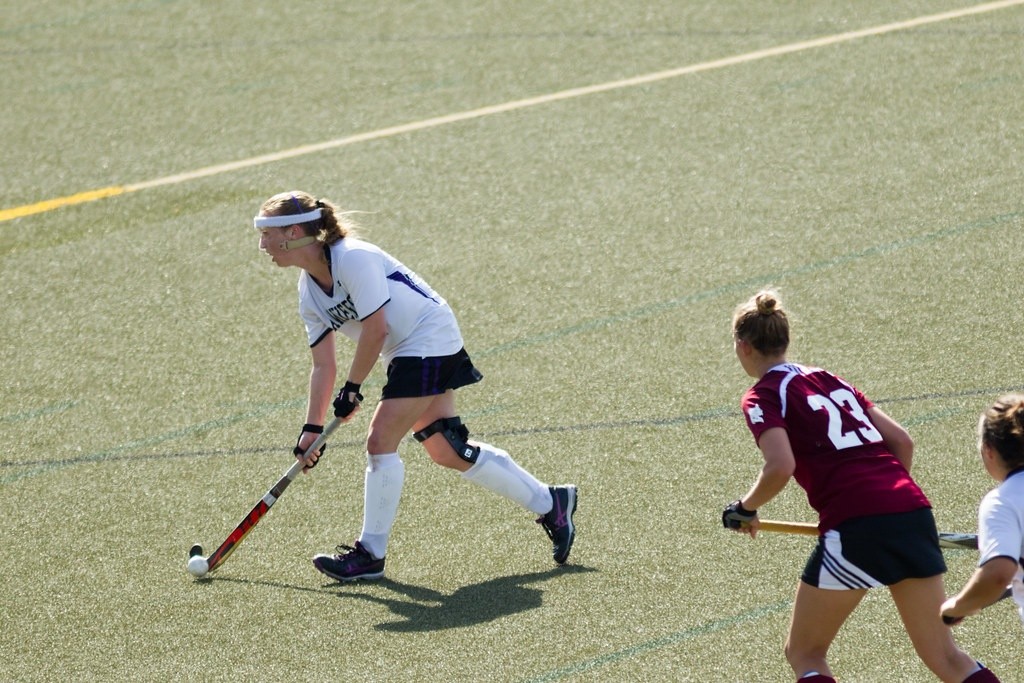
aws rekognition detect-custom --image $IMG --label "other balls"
[188,554,209,576]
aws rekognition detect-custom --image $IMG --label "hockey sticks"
[189,397,358,572]
[724,519,979,550]
[941,584,1013,624]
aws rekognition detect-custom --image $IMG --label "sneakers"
[535,484,578,564]
[313,539,385,583]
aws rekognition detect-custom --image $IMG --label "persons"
[254,190,578,582]
[940,391,1024,628]
[722,289,1000,683]
